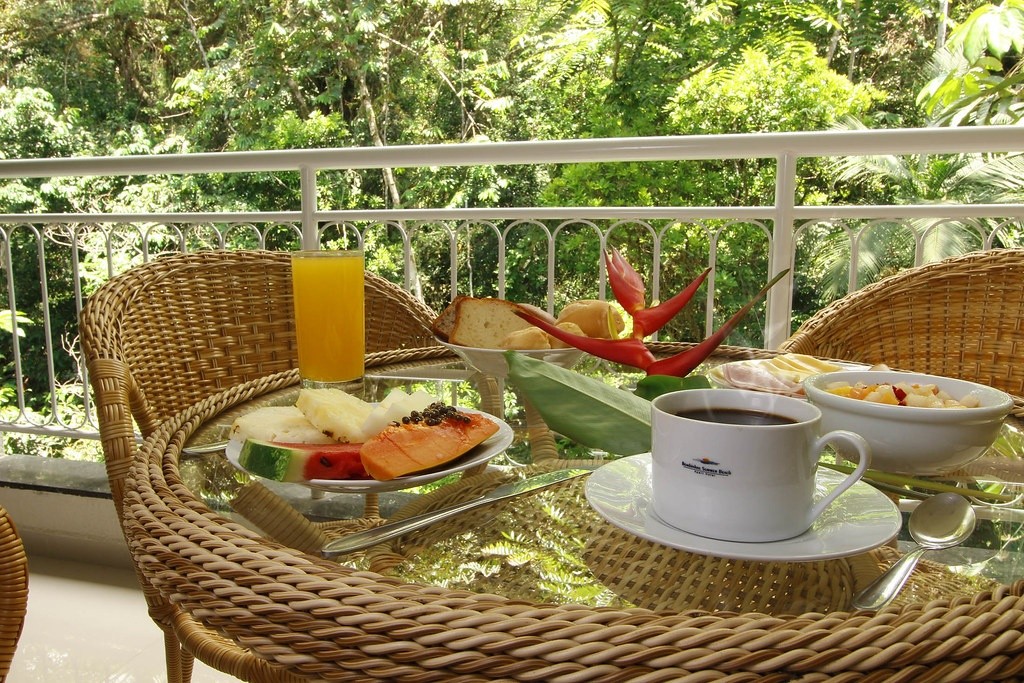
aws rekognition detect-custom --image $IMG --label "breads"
[451,294,537,351]
[554,300,624,338]
[432,293,467,340]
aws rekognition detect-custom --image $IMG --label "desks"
[130,338,1024,683]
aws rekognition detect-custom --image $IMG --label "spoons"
[852,492,976,612]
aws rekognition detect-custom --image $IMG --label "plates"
[583,452,902,562]
[709,359,872,395]
[226,402,514,493]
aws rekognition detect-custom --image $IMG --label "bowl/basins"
[432,337,584,375]
[802,371,1014,475]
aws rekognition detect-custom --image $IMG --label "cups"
[650,389,872,543]
[292,251,365,398]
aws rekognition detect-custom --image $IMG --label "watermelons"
[238,439,370,482]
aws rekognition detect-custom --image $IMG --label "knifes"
[322,468,594,553]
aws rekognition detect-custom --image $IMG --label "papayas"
[361,402,500,482]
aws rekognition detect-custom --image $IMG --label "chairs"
[79,248,507,683]
[777,248,1024,609]
